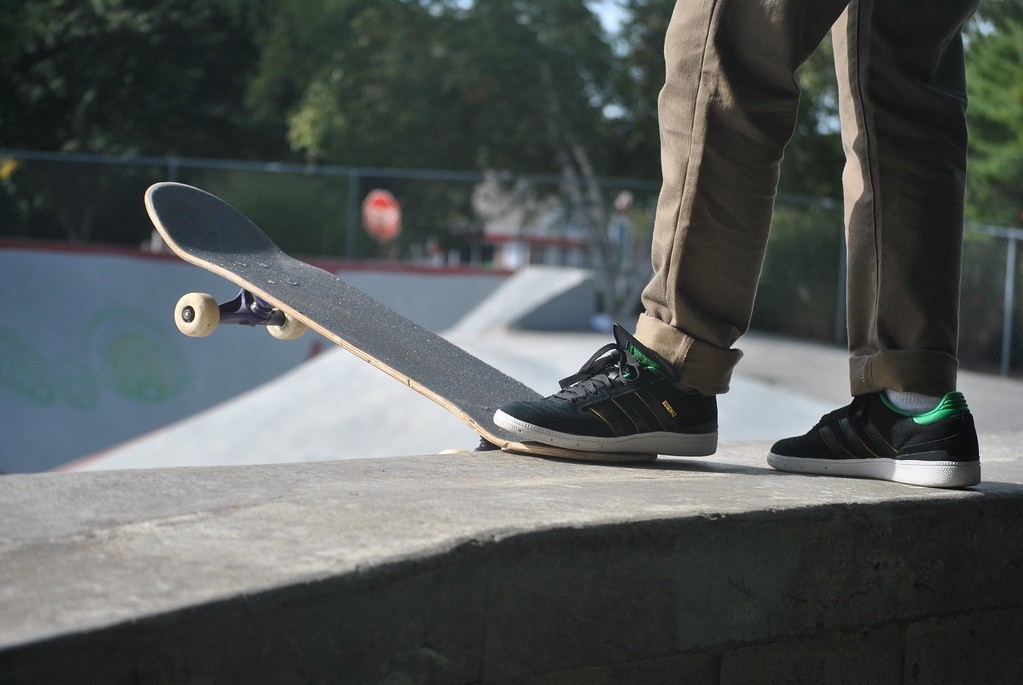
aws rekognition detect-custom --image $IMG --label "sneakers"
[768,390,981,487]
[492,322,718,457]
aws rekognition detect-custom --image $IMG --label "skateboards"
[144,180,658,466]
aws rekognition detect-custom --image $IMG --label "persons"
[493,0,989,489]
